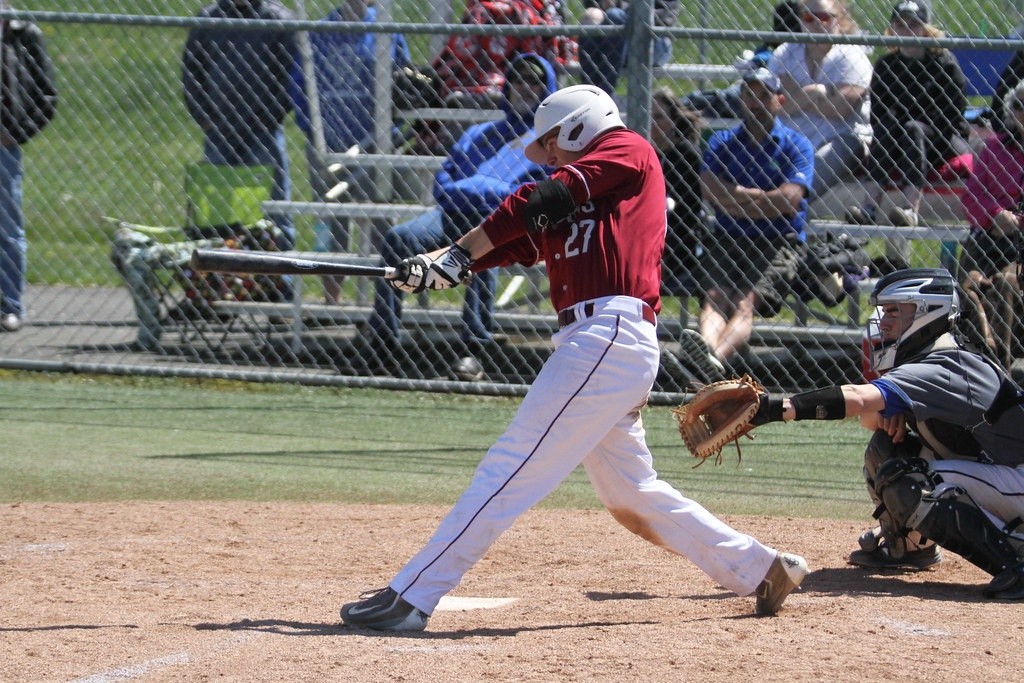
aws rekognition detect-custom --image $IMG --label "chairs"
[100,162,277,345]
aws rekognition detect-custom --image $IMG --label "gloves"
[390,254,433,295]
[425,244,475,290]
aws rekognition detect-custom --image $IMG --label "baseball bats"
[189,247,475,287]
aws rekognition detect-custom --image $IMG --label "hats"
[742,65,784,97]
[891,0,929,25]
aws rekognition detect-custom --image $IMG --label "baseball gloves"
[669,371,766,472]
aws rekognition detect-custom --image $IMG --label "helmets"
[868,266,961,374]
[522,83,629,163]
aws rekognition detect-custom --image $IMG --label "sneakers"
[341,587,427,633]
[756,552,811,617]
[848,530,941,572]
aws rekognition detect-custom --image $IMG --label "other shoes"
[680,328,726,381]
[0,313,22,331]
[844,205,874,224]
[658,349,704,394]
[336,351,402,376]
[889,205,918,227]
[450,351,485,383]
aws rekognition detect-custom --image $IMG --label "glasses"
[798,11,836,25]
[514,73,542,85]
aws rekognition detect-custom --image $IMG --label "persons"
[846,0,966,227]
[645,86,700,294]
[341,83,809,633]
[169,0,303,320]
[766,0,873,204]
[577,0,679,97]
[332,52,556,382]
[392,0,569,158]
[289,0,412,305]
[0,0,56,332]
[654,68,815,393]
[681,268,1024,598]
[956,80,1024,342]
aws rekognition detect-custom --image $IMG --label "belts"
[558,301,655,328]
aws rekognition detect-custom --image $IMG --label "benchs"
[209,61,972,346]
